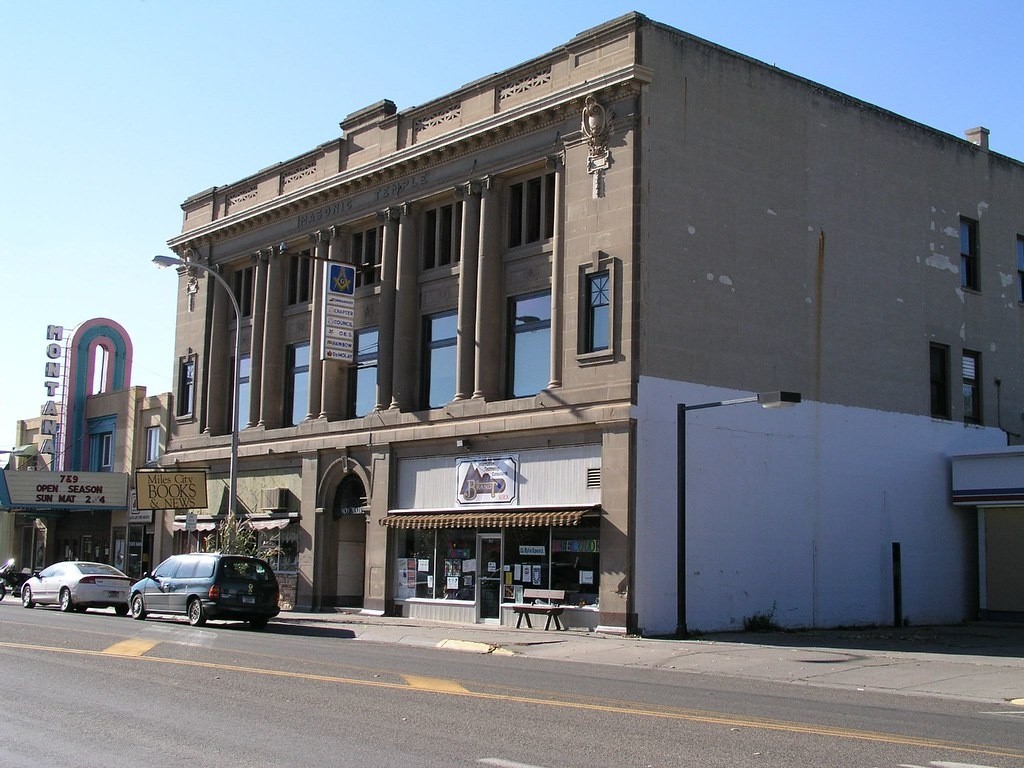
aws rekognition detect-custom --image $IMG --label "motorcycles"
[0,558,36,602]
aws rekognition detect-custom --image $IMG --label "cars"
[19,560,139,617]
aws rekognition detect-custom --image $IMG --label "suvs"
[127,550,282,632]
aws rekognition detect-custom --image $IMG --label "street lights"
[151,253,241,561]
[675,388,803,643]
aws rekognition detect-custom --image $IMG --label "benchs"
[513,607,564,631]
